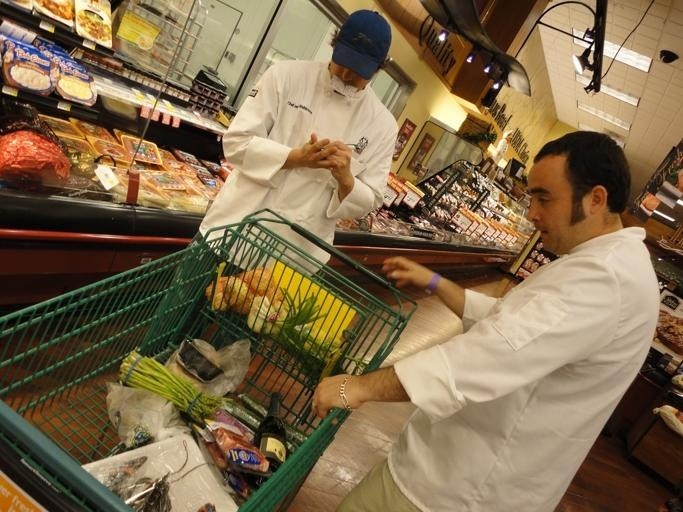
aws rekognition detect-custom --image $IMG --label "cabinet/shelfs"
[2,0,535,309]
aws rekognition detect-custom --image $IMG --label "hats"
[330,9,392,80]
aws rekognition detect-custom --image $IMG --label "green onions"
[255,287,327,357]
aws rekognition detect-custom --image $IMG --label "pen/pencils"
[321,143,360,150]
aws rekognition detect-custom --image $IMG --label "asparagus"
[118,350,224,425]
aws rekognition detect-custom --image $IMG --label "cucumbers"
[225,393,309,454]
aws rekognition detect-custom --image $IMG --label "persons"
[311,132,659,512]
[143,9,400,362]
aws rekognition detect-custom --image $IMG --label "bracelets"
[341,373,353,412]
[424,272,442,297]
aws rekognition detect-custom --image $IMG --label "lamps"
[570,0,609,95]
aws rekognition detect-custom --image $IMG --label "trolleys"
[0,206,419,511]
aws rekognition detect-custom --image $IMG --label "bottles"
[252,390,288,488]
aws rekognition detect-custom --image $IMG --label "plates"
[9,63,53,92]
[57,79,95,102]
